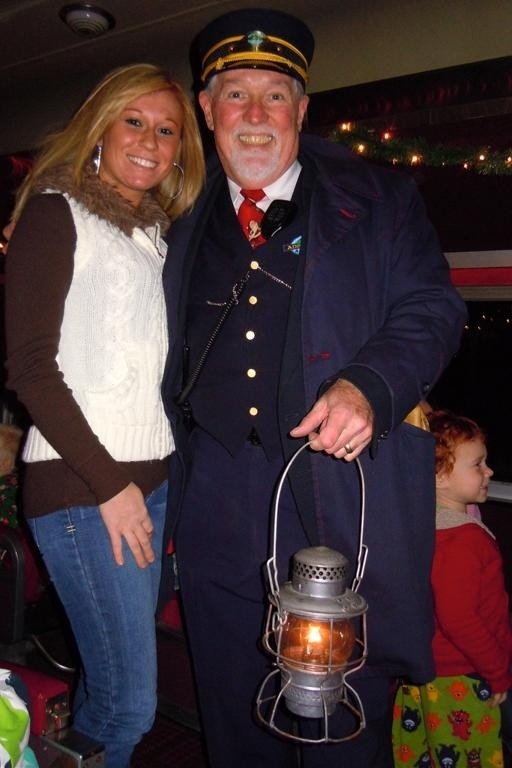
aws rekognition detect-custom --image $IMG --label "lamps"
[252,440,375,744]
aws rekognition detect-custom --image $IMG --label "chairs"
[0,513,205,768]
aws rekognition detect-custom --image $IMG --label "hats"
[197,8,314,91]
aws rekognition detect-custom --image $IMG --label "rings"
[343,443,352,455]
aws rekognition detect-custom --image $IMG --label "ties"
[235,189,266,249]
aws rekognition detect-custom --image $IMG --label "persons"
[4,57,205,765]
[1,8,469,767]
[422,407,509,712]
